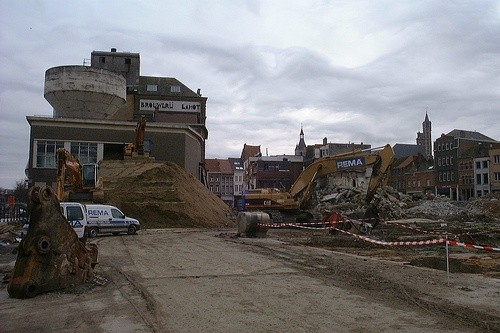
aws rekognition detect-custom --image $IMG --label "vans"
[83,204,140,237]
[21,202,88,246]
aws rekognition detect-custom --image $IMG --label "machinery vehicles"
[240,143,396,228]
[54,148,100,205]
[124,119,157,160]
